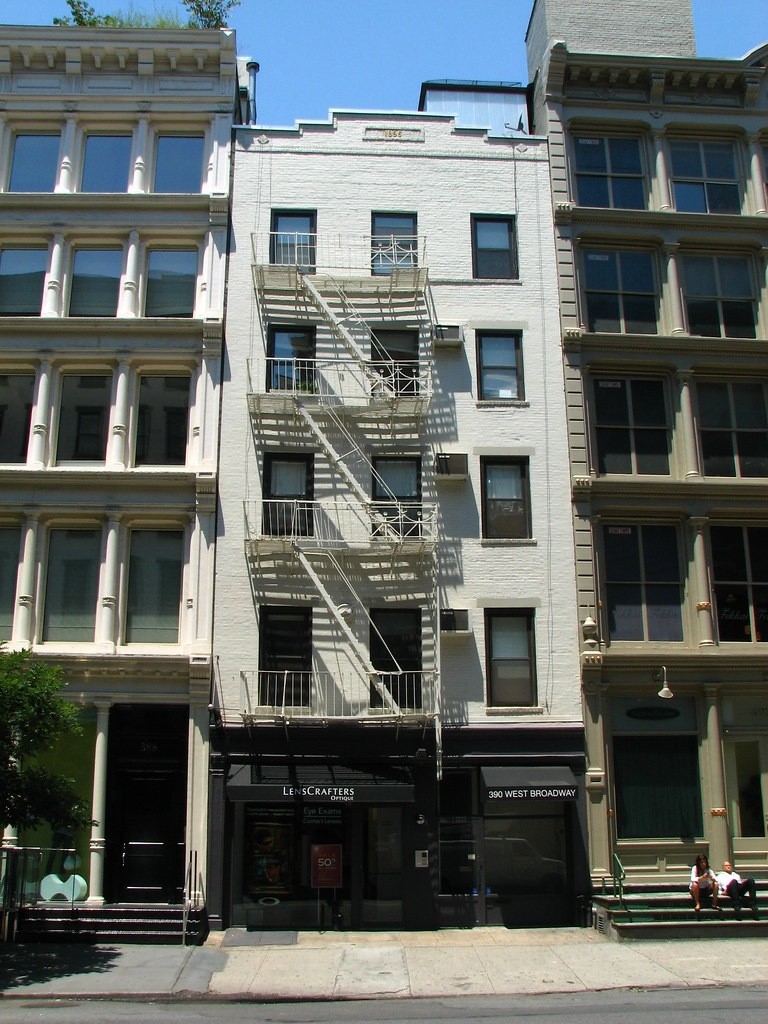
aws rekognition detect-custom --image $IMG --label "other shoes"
[750,903,759,911]
[712,905,720,909]
[734,904,742,912]
[695,905,700,911]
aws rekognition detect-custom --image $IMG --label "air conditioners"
[435,451,470,480]
[433,324,464,348]
[437,608,474,637]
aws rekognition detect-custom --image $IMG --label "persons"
[717,861,760,912]
[689,853,720,912]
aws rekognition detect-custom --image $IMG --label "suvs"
[438,837,568,897]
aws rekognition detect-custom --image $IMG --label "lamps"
[656,665,674,699]
